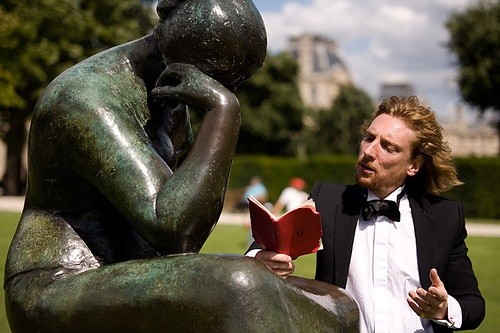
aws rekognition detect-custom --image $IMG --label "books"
[245,194,325,263]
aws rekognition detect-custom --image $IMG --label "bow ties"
[362,190,406,223]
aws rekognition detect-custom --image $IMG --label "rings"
[418,311,426,319]
[425,305,431,313]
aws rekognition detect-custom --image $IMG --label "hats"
[290,176,306,190]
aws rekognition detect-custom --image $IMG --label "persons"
[233,173,272,216]
[241,93,487,333]
[2,0,361,333]
[267,176,312,221]
